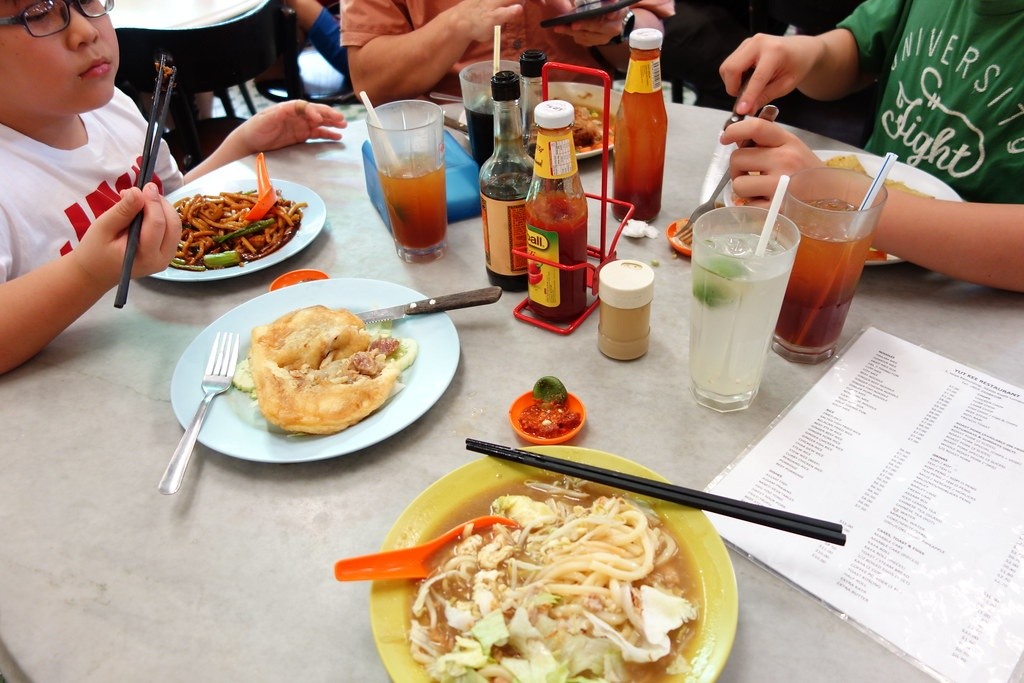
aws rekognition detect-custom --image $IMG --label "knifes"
[354,284,504,324]
[699,69,755,205]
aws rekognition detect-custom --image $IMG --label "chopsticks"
[113,54,178,308]
[465,437,847,545]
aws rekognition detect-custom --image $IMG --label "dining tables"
[0,102,1024,683]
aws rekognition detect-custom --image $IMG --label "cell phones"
[540,0,641,28]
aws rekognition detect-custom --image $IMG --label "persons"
[338,0,676,109]
[100,0,351,132]
[0,0,347,380]
[719,2,1023,295]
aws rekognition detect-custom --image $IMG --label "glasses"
[0,0,116,37]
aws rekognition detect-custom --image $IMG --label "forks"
[156,331,242,496]
[673,104,780,246]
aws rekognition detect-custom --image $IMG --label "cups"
[365,99,449,264]
[771,167,888,365]
[458,58,528,168]
[684,205,801,414]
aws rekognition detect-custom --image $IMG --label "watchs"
[613,10,635,44]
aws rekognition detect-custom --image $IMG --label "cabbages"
[425,585,698,683]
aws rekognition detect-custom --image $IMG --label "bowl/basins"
[369,444,738,683]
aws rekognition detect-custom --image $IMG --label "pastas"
[170,190,308,270]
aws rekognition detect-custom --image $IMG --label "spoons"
[335,515,521,584]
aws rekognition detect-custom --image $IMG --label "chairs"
[114,0,311,175]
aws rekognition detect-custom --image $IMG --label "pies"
[823,154,934,198]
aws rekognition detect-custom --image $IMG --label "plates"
[509,392,587,446]
[811,149,966,266]
[667,219,693,256]
[148,175,327,281]
[270,268,327,292]
[170,278,461,465]
[457,81,623,162]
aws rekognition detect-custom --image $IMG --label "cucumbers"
[363,319,419,374]
[232,359,256,392]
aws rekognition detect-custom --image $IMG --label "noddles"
[411,473,697,683]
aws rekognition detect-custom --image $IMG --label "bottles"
[475,27,667,364]
